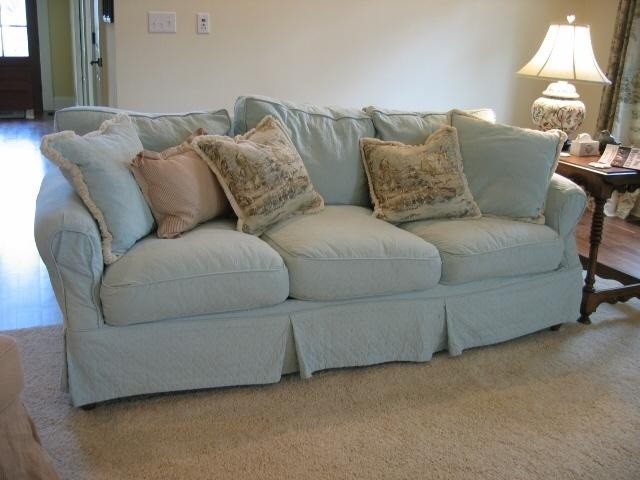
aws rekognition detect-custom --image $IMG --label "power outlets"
[197,12,209,35]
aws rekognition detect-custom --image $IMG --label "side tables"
[557,153,639,324]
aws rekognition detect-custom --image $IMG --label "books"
[598,144,640,170]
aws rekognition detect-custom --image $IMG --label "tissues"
[571,132,600,156]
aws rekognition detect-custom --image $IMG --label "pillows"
[359,124,481,226]
[192,114,324,238]
[130,128,231,239]
[41,113,156,266]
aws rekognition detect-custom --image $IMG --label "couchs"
[35,94,590,411]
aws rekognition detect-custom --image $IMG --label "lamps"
[516,13,612,144]
